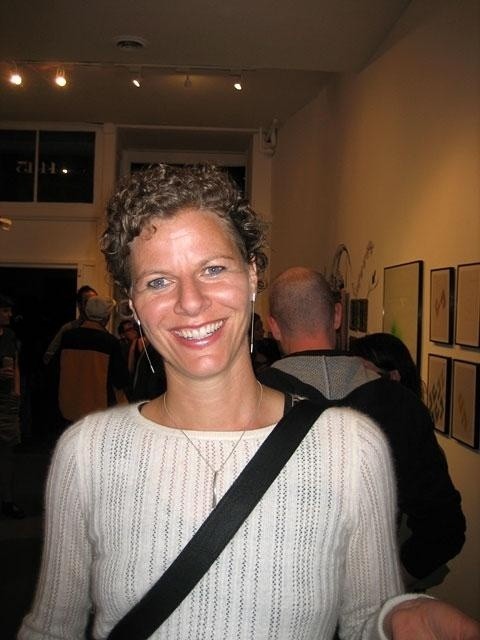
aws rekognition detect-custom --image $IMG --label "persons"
[0,296,26,520]
[15,160,480,639]
[348,332,449,594]
[249,313,280,374]
[253,266,466,593]
[40,284,168,433]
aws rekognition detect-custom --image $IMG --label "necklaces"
[163,377,264,509]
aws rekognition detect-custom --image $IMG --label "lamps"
[2,57,251,96]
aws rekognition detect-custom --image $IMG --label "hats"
[85,295,116,321]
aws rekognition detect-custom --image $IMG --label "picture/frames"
[380,259,423,380]
[450,358,479,453]
[427,351,451,434]
[454,263,480,353]
[428,265,455,348]
